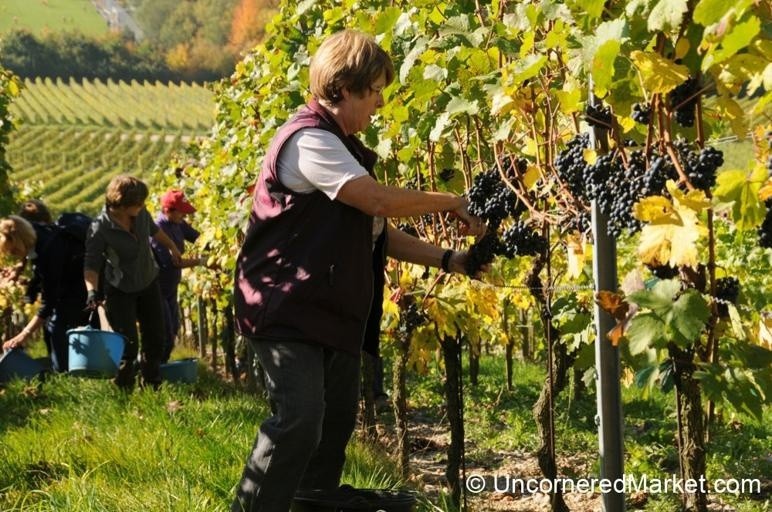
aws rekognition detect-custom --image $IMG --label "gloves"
[86,290,99,311]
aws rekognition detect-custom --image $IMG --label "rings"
[478,221,482,226]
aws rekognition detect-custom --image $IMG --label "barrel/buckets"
[0,347,49,385]
[160,358,201,386]
[64,310,128,379]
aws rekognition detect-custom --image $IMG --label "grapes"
[398,77,772,334]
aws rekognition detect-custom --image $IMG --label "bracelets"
[441,249,452,273]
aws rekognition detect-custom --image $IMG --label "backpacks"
[40,212,91,273]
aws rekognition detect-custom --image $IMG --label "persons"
[0,215,84,374]
[139,189,223,364]
[231,31,498,511]
[83,176,184,408]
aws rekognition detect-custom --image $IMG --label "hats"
[160,190,196,214]
